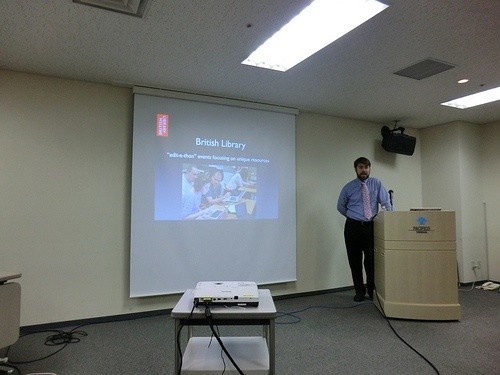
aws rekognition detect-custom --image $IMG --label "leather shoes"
[367,287,374,300]
[353,290,365,301]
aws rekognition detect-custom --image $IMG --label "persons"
[182,163,250,220]
[337,157,392,302]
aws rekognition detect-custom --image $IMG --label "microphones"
[388,189,393,206]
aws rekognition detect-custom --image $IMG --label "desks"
[0,270,21,350]
[237,172,256,199]
[170,287,278,375]
[192,198,256,218]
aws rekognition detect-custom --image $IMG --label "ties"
[360,182,372,220]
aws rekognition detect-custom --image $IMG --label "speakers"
[382,133,416,156]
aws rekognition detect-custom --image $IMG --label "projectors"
[193,281,259,308]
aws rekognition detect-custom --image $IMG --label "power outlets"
[473,264,483,273]
